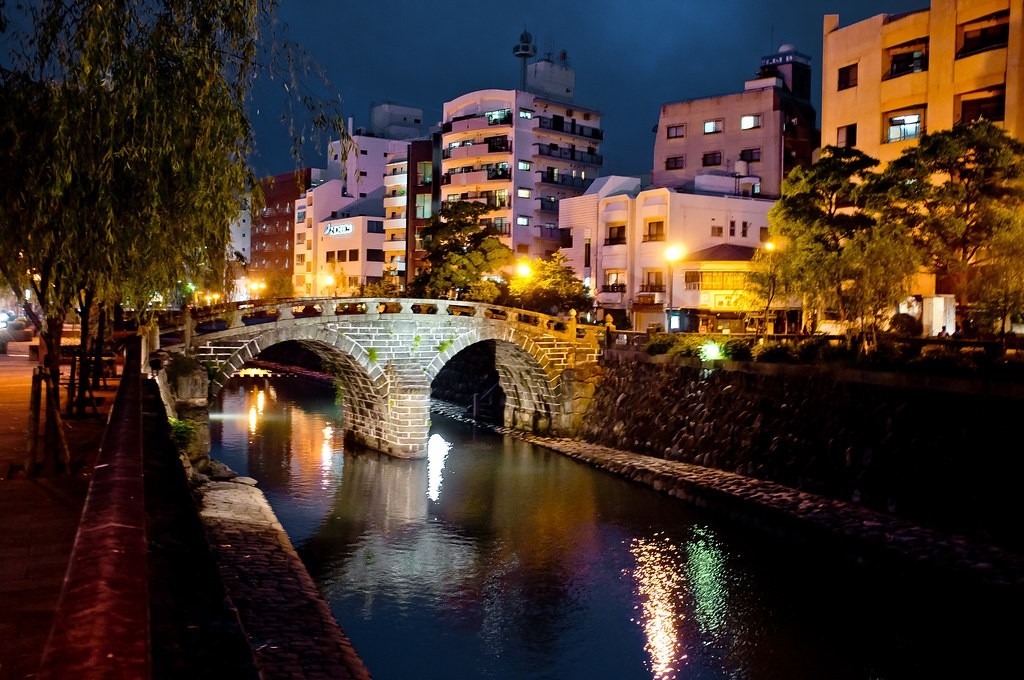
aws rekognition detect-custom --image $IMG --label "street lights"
[765,242,775,341]
[325,276,333,299]
[518,262,531,319]
[197,279,267,305]
[666,245,682,331]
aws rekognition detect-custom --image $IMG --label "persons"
[787,322,796,334]
[955,320,961,334]
[937,325,950,348]
